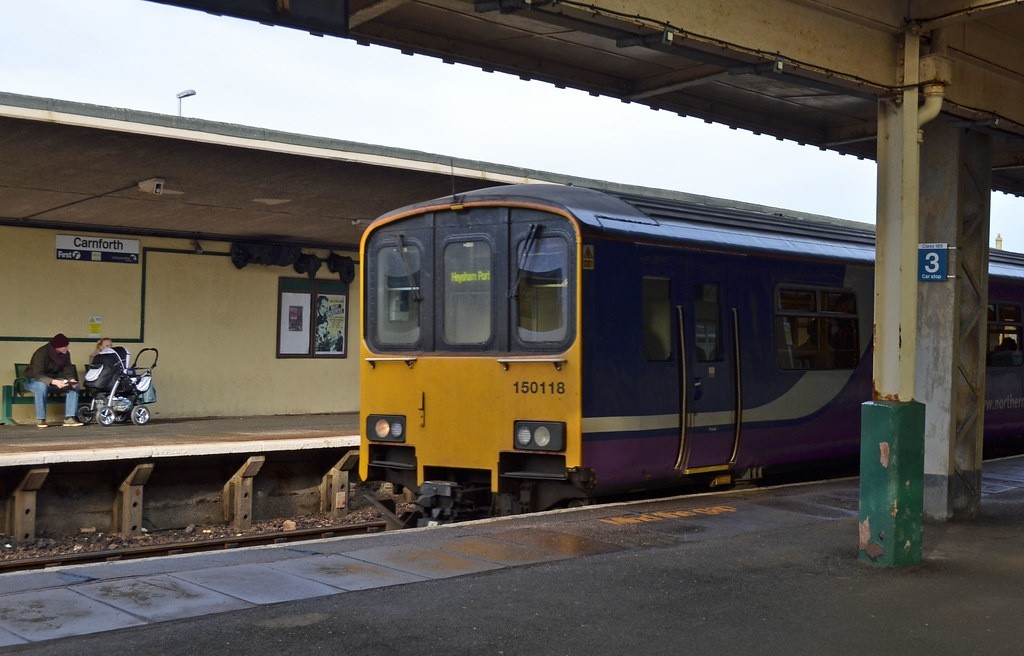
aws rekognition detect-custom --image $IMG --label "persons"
[23,333,84,428]
[88,338,113,364]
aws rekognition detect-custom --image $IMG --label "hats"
[51,333,68,347]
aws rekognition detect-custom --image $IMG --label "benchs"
[0,363,94,427]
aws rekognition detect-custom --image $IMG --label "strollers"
[74,344,159,427]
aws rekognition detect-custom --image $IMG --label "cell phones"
[65,382,77,385]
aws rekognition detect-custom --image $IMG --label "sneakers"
[62,418,83,427]
[37,420,49,428]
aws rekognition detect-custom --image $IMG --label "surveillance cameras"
[138,178,165,195]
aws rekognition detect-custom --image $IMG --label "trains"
[359,157,1024,513]
